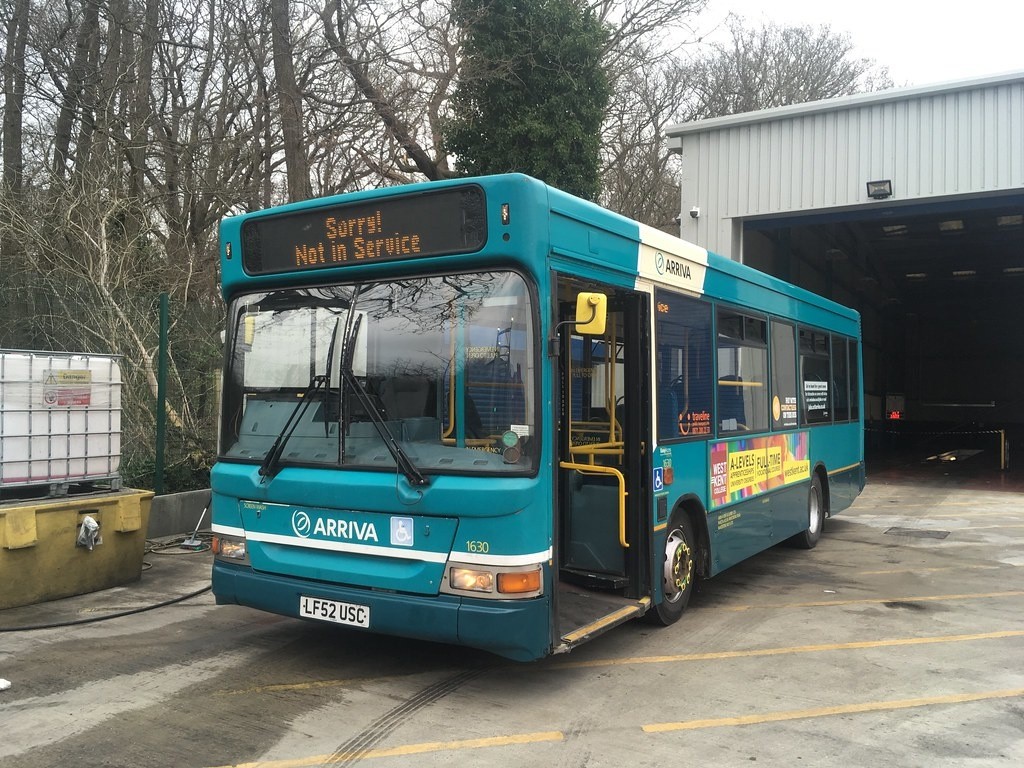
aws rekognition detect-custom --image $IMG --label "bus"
[213,173,864,663]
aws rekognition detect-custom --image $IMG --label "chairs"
[585,371,839,465]
[442,343,527,436]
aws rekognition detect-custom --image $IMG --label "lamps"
[866,179,893,200]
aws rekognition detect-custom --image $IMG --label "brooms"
[178,492,212,551]
